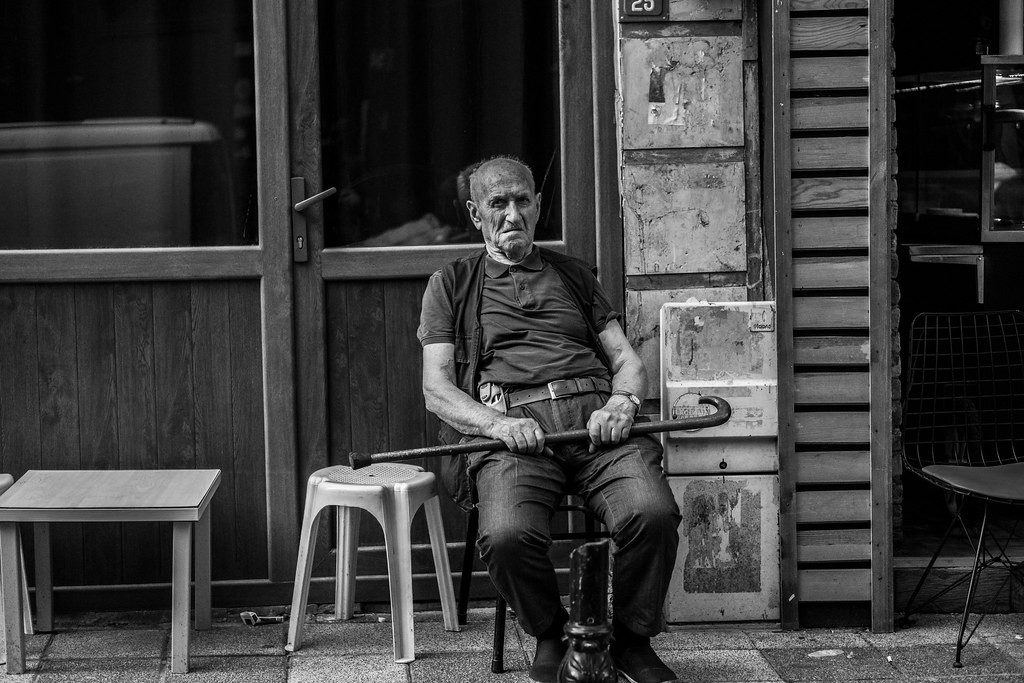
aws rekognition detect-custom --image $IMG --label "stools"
[284,463,460,663]
[460,484,610,673]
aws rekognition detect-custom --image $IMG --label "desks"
[0,469,225,675]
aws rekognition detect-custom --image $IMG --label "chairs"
[903,307,1024,670]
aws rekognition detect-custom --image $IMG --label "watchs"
[611,389,641,413]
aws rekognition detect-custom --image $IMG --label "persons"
[417,153,683,683]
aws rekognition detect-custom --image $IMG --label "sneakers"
[528,625,572,683]
[609,620,677,683]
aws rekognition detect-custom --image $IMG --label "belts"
[504,377,614,408]
